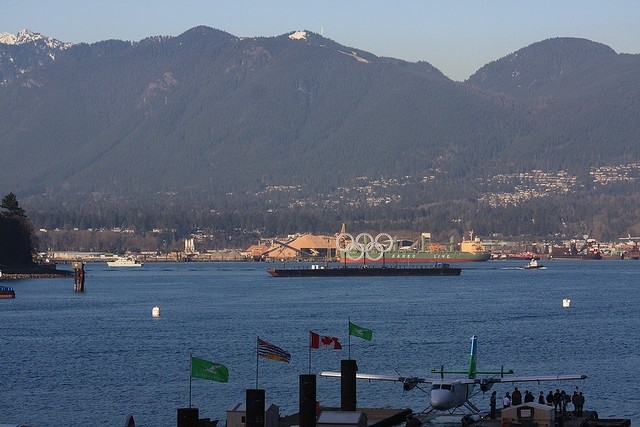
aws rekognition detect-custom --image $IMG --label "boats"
[1,286,16,299]
[107,254,144,267]
[524,259,543,269]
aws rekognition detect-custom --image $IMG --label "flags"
[258,338,291,363]
[311,332,342,350]
[191,357,229,382]
[349,321,372,341]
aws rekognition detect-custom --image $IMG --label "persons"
[489,391,497,419]
[571,391,579,412]
[512,387,522,405]
[553,389,562,413]
[539,391,546,405]
[561,389,567,413]
[524,390,535,403]
[503,395,511,409]
[546,391,554,407]
[578,392,585,415]
[504,392,511,401]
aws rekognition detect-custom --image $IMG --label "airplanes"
[320,334,587,427]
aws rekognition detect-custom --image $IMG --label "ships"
[266,267,461,277]
[338,235,492,263]
